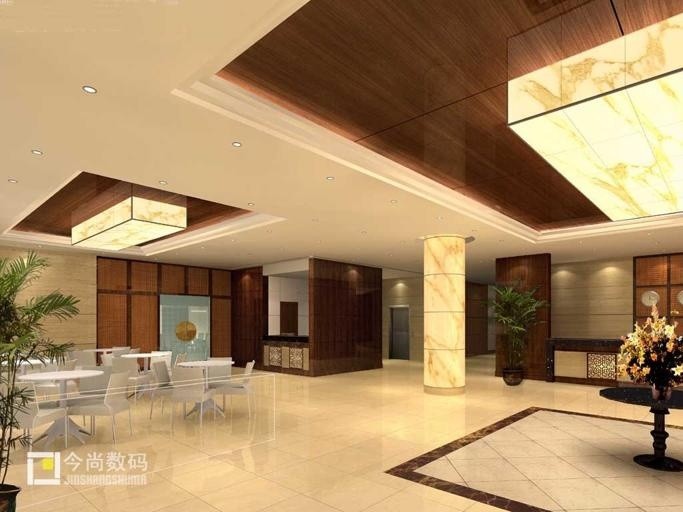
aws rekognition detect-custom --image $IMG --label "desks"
[600,387,683,471]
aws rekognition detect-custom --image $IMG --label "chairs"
[6,346,256,452]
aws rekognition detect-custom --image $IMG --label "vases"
[651,384,671,401]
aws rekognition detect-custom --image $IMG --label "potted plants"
[0,249,81,512]
[483,279,550,386]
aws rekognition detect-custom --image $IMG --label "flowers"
[615,303,683,389]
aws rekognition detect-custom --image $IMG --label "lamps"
[71,196,187,252]
[506,12,682,221]
